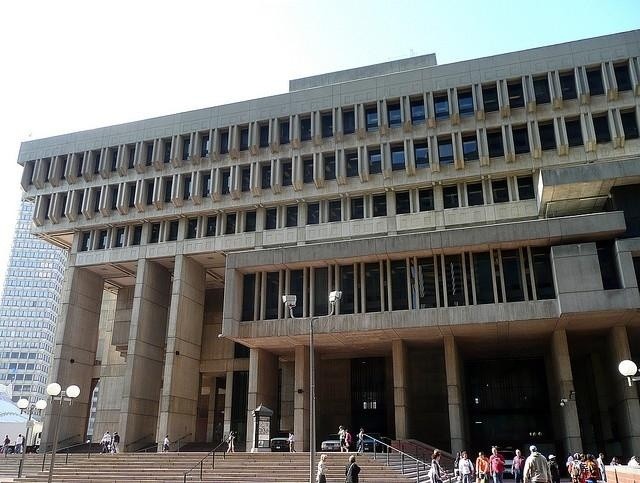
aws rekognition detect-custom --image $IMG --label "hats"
[548,454,557,460]
[529,445,537,453]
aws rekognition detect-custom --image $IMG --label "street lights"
[282,291,344,482]
[47,381,82,483]
[17,397,47,477]
[618,359,640,387]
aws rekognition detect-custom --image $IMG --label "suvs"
[497,450,518,477]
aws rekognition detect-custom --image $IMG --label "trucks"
[0,421,44,454]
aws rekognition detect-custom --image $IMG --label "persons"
[337,425,349,452]
[357,427,364,452]
[13,434,23,454]
[288,432,297,452]
[345,454,361,483]
[98,430,120,454]
[1,434,10,452]
[163,434,170,452]
[345,429,351,451]
[428,444,640,482]
[226,430,235,453]
[316,453,329,483]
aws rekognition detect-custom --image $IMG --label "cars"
[355,432,383,452]
[270,437,290,451]
[321,433,341,451]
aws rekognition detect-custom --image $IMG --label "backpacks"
[549,463,557,478]
[568,460,600,480]
